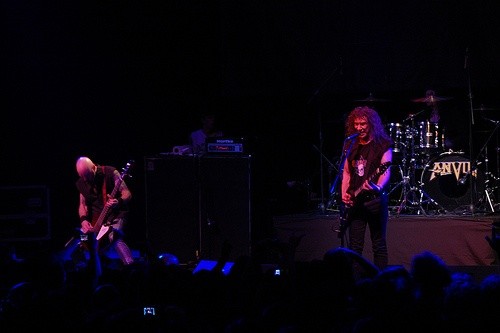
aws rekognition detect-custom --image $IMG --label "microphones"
[346,132,362,140]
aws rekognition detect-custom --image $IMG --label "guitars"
[91,162,133,240]
[338,160,393,237]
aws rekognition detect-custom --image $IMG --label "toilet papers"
[173,145,186,154]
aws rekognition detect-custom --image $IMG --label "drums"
[385,121,412,153]
[406,149,486,210]
[409,122,439,149]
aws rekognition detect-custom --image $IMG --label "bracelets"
[80,216,87,222]
[118,198,123,206]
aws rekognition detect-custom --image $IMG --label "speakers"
[139,154,251,264]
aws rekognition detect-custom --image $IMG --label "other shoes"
[96,283,105,298]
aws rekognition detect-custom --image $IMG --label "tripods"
[388,43,500,217]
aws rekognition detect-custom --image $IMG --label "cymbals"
[473,107,497,110]
[353,97,387,101]
[411,95,448,102]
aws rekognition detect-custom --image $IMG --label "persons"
[340,105,394,266]
[409,87,446,129]
[0,227,500,333]
[76,156,133,264]
